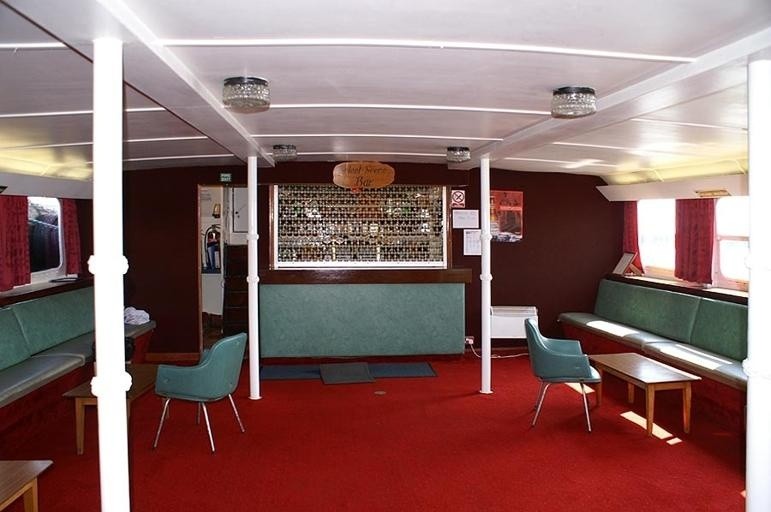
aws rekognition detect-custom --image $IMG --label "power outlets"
[465,335,475,345]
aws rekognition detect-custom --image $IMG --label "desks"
[582,349,704,440]
[0,458,54,511]
[58,360,170,455]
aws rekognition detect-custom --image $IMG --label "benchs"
[638,296,748,434]
[8,284,157,383]
[552,275,703,389]
[0,306,83,409]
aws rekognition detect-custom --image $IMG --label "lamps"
[221,74,272,115]
[272,144,298,163]
[445,144,472,165]
[549,85,599,121]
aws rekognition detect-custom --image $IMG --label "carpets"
[318,361,377,387]
[259,359,439,382]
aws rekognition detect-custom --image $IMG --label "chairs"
[522,316,604,434]
[149,331,249,455]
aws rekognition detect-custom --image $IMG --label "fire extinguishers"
[204,224,221,274]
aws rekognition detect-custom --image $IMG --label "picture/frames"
[611,251,639,276]
